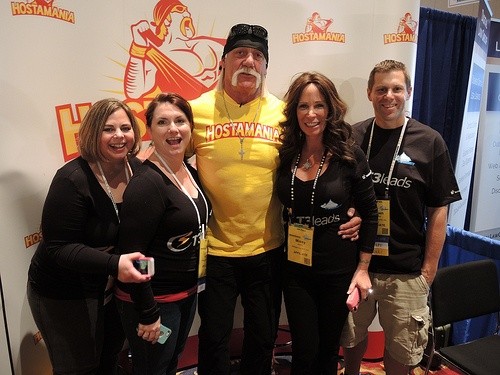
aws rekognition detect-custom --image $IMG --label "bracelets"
[359,259,370,265]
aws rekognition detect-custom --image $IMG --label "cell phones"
[346,286,361,312]
[132,256,155,278]
[148,324,172,345]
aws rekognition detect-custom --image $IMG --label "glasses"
[230,23,268,39]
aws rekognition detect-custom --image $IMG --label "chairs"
[425,259,500,375]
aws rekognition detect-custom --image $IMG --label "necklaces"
[222,88,264,159]
[96,158,133,224]
[302,155,311,171]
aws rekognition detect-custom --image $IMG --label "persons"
[27,99,151,375]
[351,60,462,375]
[143,23,361,375]
[274,70,378,375]
[112,93,212,375]
